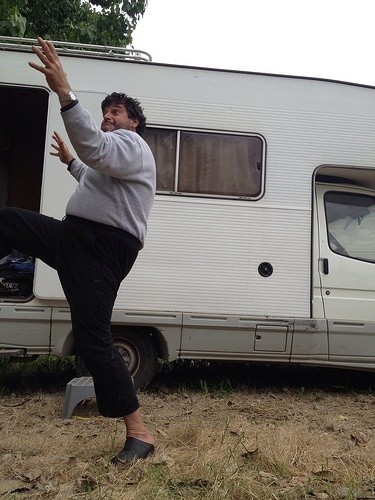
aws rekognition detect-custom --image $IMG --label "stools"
[61,376,96,419]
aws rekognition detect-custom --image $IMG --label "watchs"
[59,90,76,101]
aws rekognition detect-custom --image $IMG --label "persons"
[0,37,156,464]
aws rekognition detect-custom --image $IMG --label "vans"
[0,35,375,391]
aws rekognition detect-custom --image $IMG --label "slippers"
[111,436,155,466]
